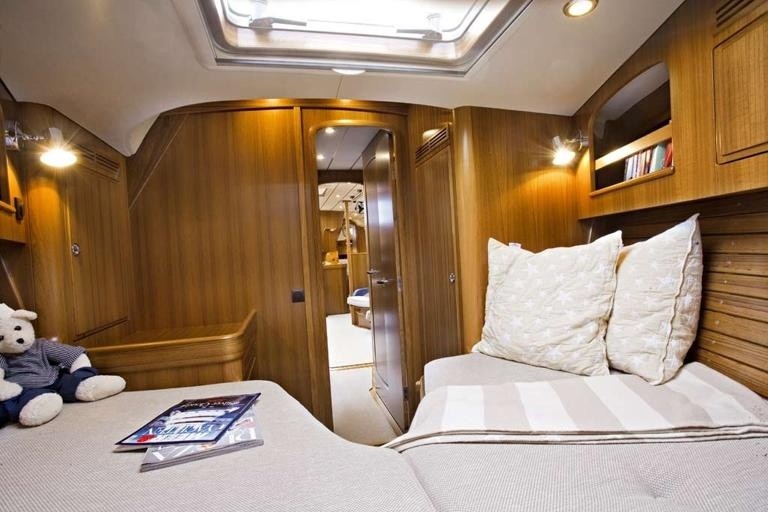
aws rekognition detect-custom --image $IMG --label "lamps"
[6,118,78,168]
[551,129,589,166]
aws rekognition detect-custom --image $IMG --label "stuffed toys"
[0,303,125,427]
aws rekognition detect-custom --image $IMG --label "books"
[115,393,260,452]
[623,139,672,182]
[140,403,264,472]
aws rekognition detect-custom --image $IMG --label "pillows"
[603,212,704,386]
[471,229,623,376]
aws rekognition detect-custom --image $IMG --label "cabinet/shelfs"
[572,0,768,221]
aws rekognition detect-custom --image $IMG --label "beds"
[0,378,435,512]
[401,352,768,512]
